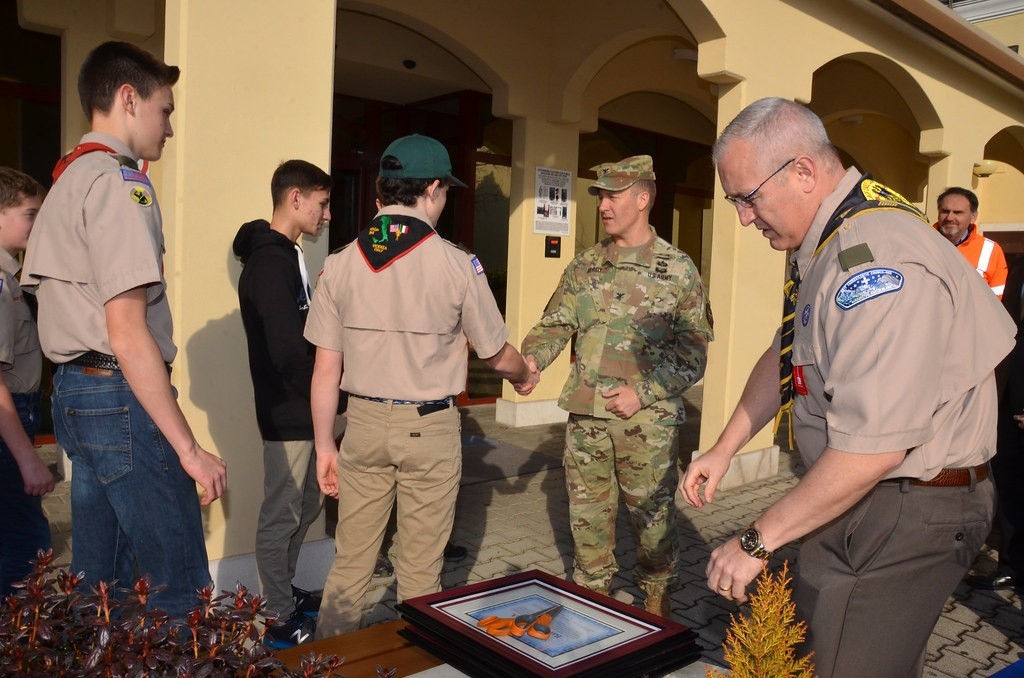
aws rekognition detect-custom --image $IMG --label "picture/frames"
[394,569,705,678]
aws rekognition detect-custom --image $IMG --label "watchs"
[740,521,773,561]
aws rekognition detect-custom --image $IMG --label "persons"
[232,160,338,650]
[679,96,1018,678]
[519,156,715,620]
[963,253,1024,600]
[933,186,1006,305]
[21,41,228,632]
[303,134,541,638]
[0,167,56,602]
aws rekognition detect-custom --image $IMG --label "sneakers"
[263,607,317,650]
[291,583,322,617]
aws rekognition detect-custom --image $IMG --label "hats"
[379,133,470,188]
[586,155,656,197]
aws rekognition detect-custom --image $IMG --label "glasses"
[724,159,795,208]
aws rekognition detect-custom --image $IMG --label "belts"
[347,390,457,417]
[66,351,173,375]
[876,464,992,487]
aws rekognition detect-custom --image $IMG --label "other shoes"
[966,545,1000,590]
[442,542,468,561]
[642,593,674,619]
[371,551,394,577]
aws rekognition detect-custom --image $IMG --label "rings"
[718,585,731,591]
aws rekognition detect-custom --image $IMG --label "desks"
[241,618,731,678]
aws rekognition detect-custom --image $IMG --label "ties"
[772,173,931,453]
[52,143,118,184]
[357,215,435,272]
